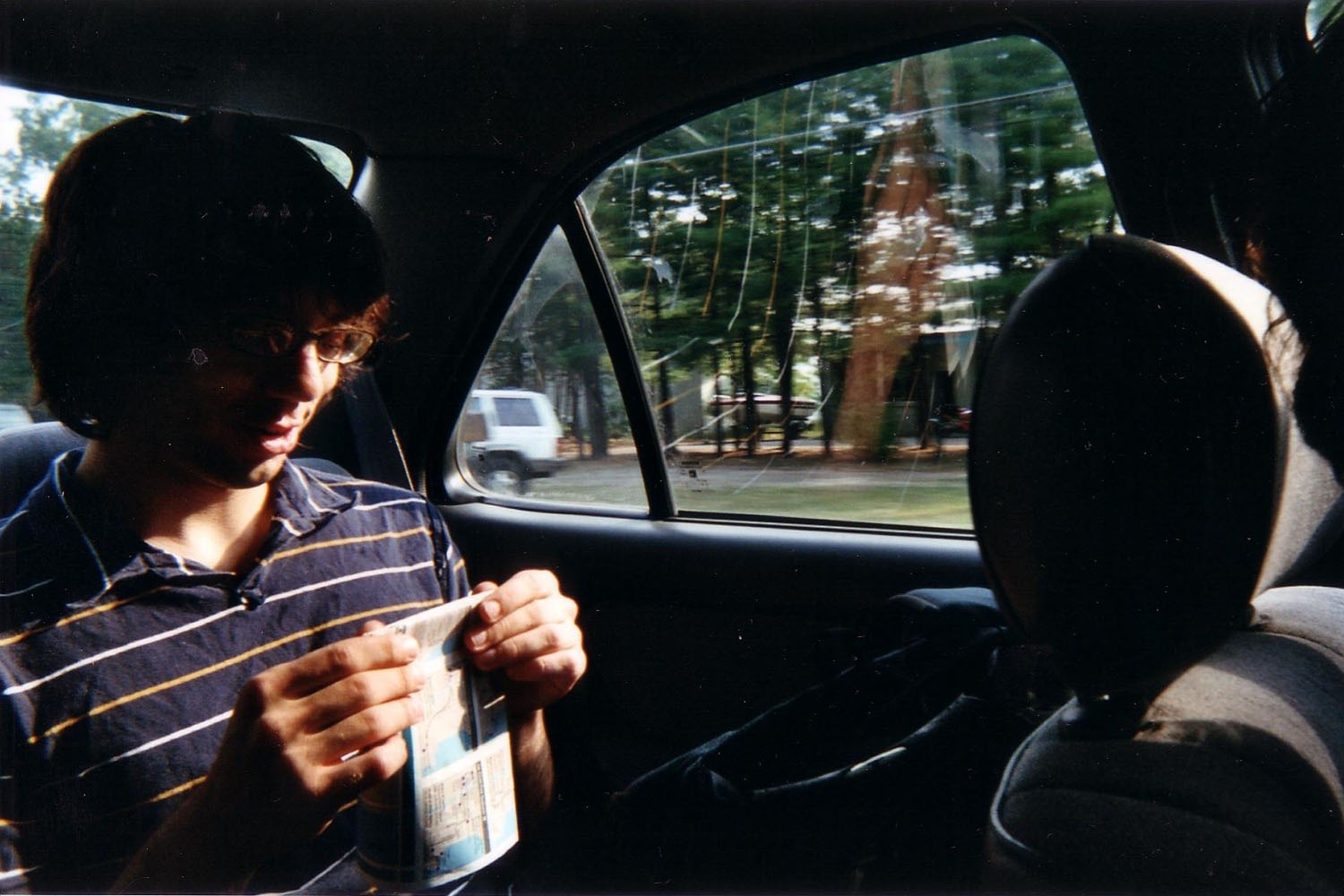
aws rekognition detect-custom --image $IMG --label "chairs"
[966,236,1344,896]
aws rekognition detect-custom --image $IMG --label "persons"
[1229,1,1344,487]
[0,112,587,895]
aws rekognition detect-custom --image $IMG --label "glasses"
[235,320,377,366]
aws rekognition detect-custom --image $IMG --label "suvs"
[460,389,568,497]
